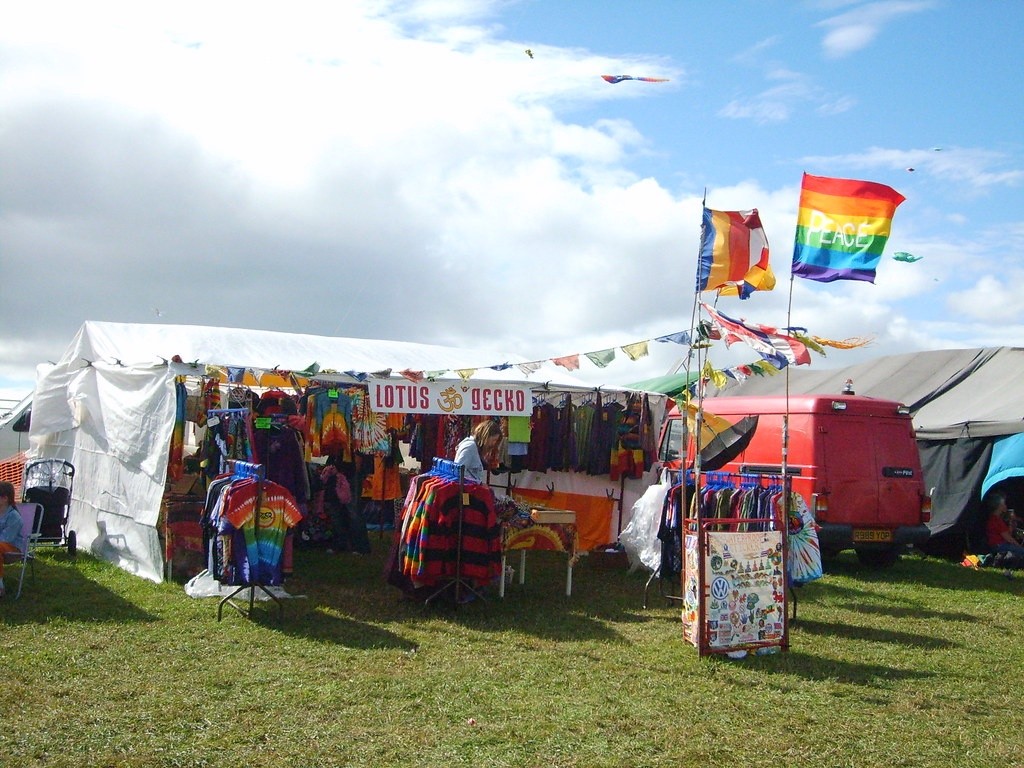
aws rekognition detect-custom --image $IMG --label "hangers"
[426,456,477,486]
[228,458,269,484]
[673,468,793,494]
[532,388,650,411]
[176,375,368,431]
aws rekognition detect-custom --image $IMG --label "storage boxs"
[528,505,577,523]
[505,568,514,584]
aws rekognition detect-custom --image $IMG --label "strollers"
[18,459,79,555]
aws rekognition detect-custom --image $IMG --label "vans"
[656,395,934,568]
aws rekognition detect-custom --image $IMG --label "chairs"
[3,502,45,600]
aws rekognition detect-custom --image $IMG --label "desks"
[498,519,577,598]
[154,499,207,584]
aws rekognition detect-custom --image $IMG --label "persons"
[984,490,1020,549]
[0,481,23,597]
[454,420,503,484]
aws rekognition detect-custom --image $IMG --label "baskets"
[505,565,515,584]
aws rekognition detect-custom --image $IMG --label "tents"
[621,346,1024,563]
[0,392,33,462]
[25,321,668,583]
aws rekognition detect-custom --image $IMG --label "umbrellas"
[687,414,758,471]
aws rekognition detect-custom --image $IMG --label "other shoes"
[0,577,4,589]
[1004,551,1013,560]
[994,552,1004,560]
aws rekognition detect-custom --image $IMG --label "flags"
[696,208,776,300]
[792,173,906,284]
